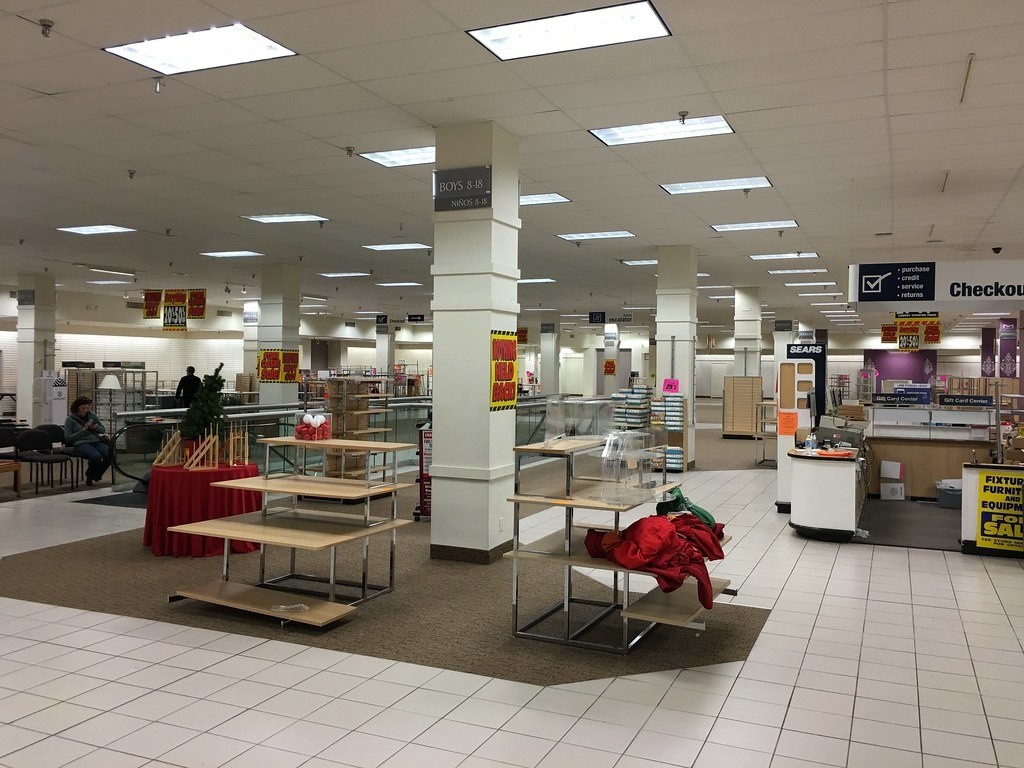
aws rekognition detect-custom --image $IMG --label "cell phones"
[92,422,99,425]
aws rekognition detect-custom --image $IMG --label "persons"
[176,366,203,408]
[299,370,303,399]
[306,374,314,401]
[65,396,117,486]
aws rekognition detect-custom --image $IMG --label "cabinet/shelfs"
[864,406,997,442]
[1001,395,1024,421]
[753,401,777,470]
[299,360,432,481]
[995,382,1024,465]
[947,377,1005,397]
[651,399,688,471]
[166,433,415,631]
[65,370,158,443]
[830,368,877,403]
[502,435,737,656]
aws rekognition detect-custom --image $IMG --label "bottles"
[811,433,816,449]
[805,435,812,456]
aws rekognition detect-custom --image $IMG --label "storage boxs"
[236,372,259,403]
[295,413,333,441]
[936,485,962,509]
[952,380,1024,465]
[611,385,684,471]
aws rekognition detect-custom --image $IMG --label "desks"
[218,391,258,398]
[143,461,263,558]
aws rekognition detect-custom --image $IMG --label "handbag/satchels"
[100,435,111,446]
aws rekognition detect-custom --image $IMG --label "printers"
[816,416,865,448]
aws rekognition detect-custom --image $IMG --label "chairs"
[0,424,89,495]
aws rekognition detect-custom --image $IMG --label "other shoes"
[92,480,100,486]
[85,477,93,490]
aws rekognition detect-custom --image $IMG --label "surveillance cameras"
[992,247,1003,254]
